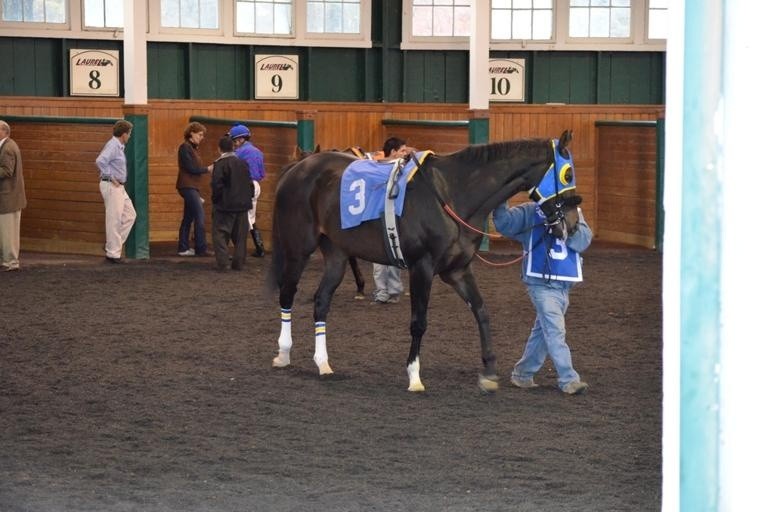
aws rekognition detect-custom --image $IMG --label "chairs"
[195,250,215,257]
[510,377,538,388]
[178,249,195,256]
[560,382,587,395]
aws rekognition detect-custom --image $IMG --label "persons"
[210,136,254,271]
[493,198,592,395]
[226,125,265,257]
[94,121,138,264]
[0,120,27,273]
[370,138,409,305]
[174,122,214,257]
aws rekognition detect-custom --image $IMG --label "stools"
[101,178,110,180]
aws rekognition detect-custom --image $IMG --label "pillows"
[230,125,250,139]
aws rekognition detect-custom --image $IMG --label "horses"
[295,145,418,305]
[269,130,580,394]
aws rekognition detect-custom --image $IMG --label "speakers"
[250,226,264,256]
